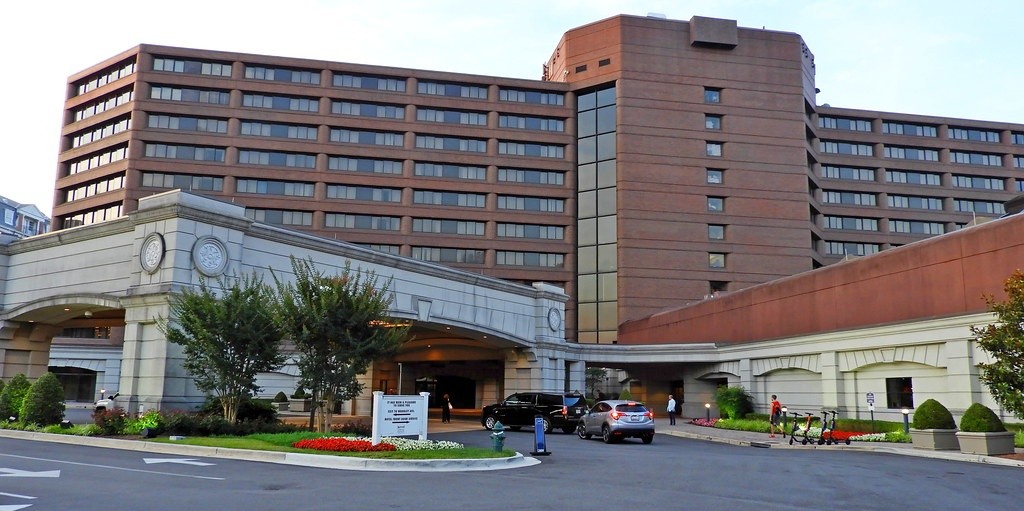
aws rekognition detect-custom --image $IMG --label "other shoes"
[443,421,446,424]
[669,424,672,426]
[448,421,451,424]
[672,424,675,426]
[769,435,775,438]
[782,432,786,438]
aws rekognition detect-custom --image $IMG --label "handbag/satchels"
[448,399,453,410]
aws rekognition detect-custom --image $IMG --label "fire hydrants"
[488,420,507,452]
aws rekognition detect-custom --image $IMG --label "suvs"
[480,391,590,434]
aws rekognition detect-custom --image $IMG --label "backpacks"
[775,407,781,417]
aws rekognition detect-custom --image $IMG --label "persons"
[769,395,786,438]
[667,395,676,425]
[597,390,605,400]
[442,394,451,423]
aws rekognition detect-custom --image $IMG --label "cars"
[576,399,656,444]
[92,391,120,415]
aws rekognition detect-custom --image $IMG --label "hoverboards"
[816,410,833,445]
[789,411,809,445]
[801,411,819,445]
[825,410,851,445]
